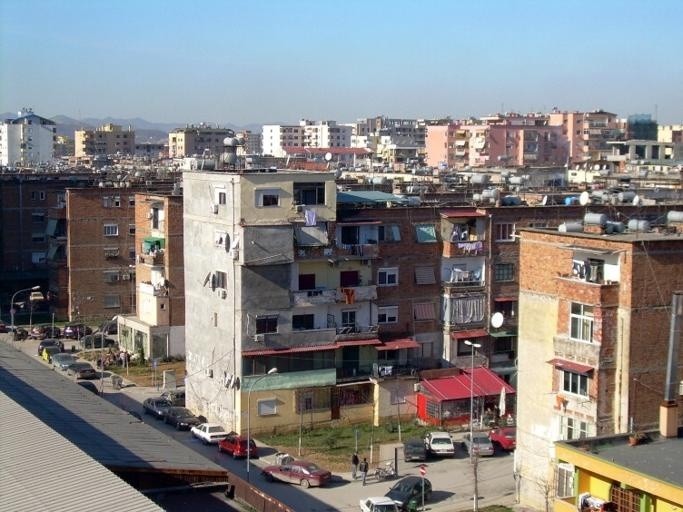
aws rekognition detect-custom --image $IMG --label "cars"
[384,476,433,509]
[261,458,333,489]
[162,405,201,431]
[0,319,120,399]
[358,496,399,512]
[141,395,174,421]
[11,289,57,312]
[188,422,230,446]
[402,425,515,463]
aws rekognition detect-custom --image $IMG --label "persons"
[359,458,368,487]
[96,349,130,371]
[351,450,358,481]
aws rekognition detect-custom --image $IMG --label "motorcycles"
[372,460,397,482]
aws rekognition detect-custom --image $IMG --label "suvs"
[159,390,186,408]
[215,433,257,459]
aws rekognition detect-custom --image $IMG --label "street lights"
[8,284,42,349]
[246,365,278,485]
[463,339,481,464]
[98,315,122,397]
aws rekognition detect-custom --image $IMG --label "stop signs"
[419,466,425,476]
